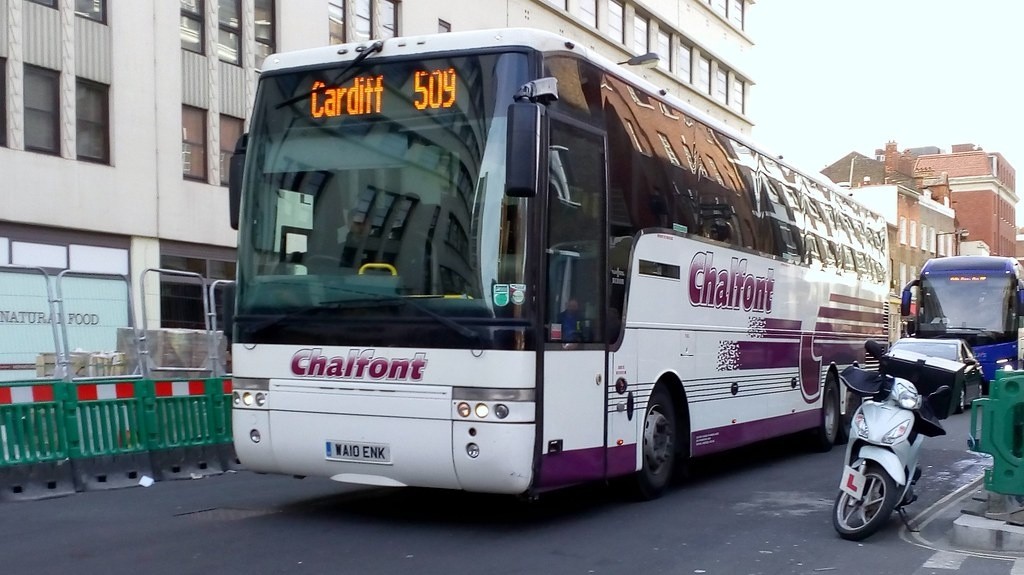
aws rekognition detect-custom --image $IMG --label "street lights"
[935,229,970,258]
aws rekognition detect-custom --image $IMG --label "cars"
[889,337,985,414]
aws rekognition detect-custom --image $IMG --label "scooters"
[832,338,952,543]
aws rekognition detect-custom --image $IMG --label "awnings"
[903,303,915,315]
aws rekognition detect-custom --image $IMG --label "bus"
[228,26,892,501]
[899,255,1024,394]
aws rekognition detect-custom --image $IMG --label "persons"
[287,192,469,266]
